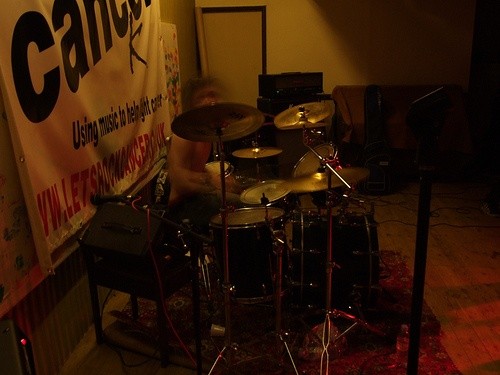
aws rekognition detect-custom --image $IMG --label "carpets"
[105,249,462,375]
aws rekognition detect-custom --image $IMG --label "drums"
[204,161,236,185]
[290,208,380,317]
[209,207,288,304]
[240,179,297,212]
[290,142,344,179]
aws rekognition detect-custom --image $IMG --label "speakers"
[77,203,175,274]
[276,121,329,180]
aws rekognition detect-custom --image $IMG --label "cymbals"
[280,122,329,130]
[274,102,331,128]
[232,147,283,159]
[171,102,265,142]
[281,166,370,193]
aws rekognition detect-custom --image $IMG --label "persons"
[161,76,277,310]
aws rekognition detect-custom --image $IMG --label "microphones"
[88,192,136,206]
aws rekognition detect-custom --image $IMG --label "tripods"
[206,126,390,375]
